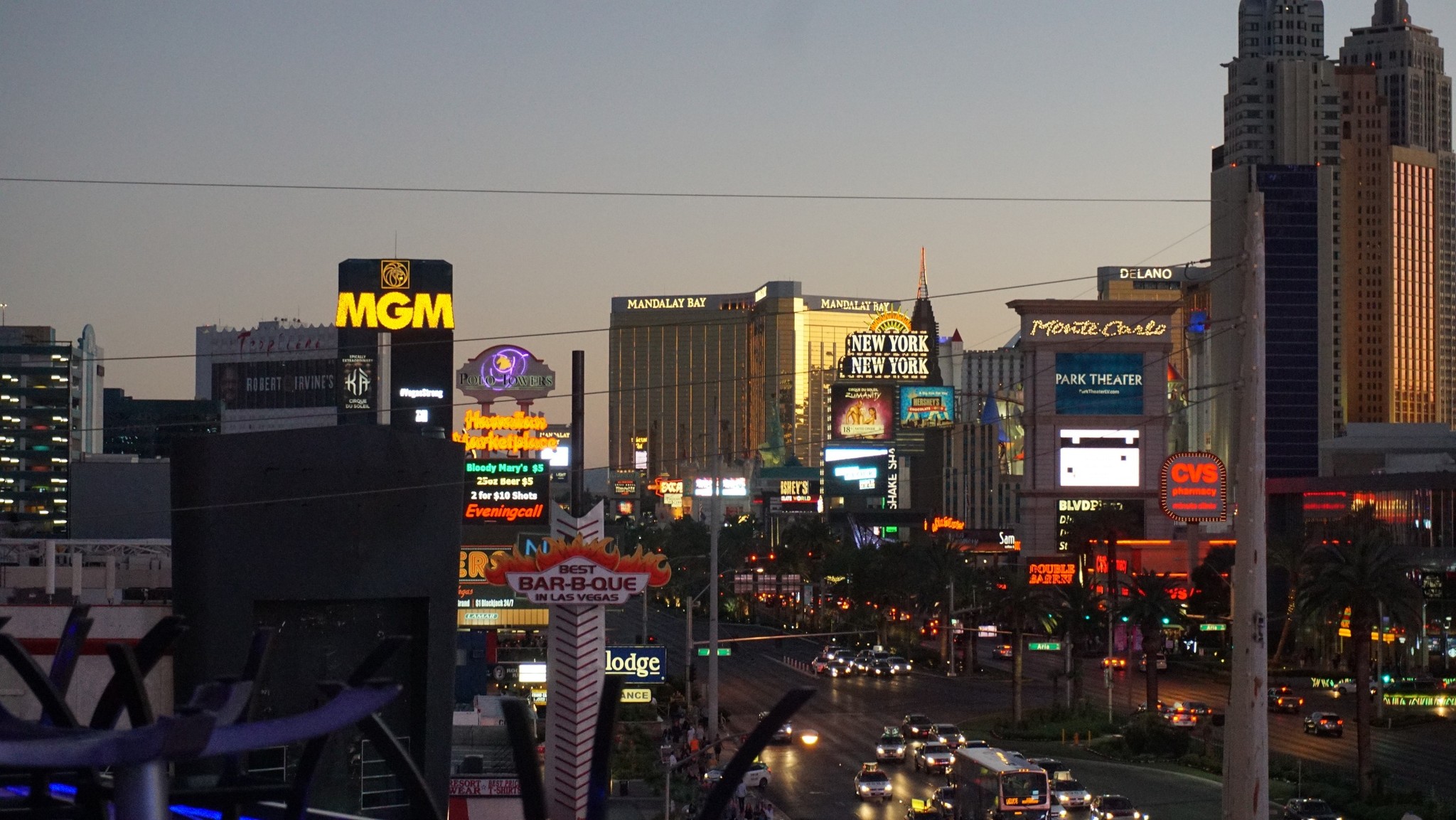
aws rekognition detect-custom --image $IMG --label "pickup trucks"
[1268,687,1303,715]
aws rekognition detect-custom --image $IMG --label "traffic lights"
[648,634,655,647]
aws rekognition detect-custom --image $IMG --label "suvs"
[914,742,953,775]
[928,723,966,749]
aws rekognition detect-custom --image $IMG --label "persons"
[662,693,775,820]
[499,634,548,661]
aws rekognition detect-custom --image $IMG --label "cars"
[992,645,1014,661]
[1283,798,1341,820]
[811,645,912,679]
[1332,675,1456,706]
[758,712,792,745]
[904,741,1091,820]
[902,714,933,739]
[854,762,892,800]
[1303,711,1342,738]
[1136,700,1212,730]
[874,726,907,764]
[703,761,772,790]
[1089,795,1140,820]
[1100,653,1167,674]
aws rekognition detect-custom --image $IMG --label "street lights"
[665,729,818,820]
[685,568,764,720]
[642,554,705,644]
